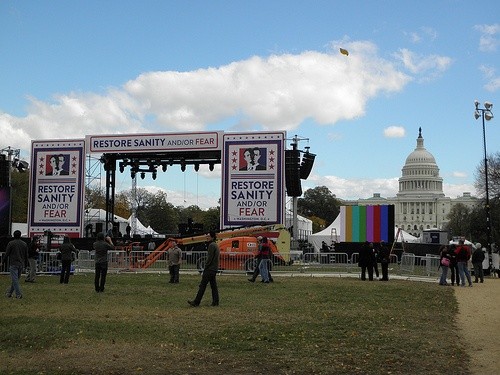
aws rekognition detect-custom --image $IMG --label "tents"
[84,208,165,238]
[307,211,339,258]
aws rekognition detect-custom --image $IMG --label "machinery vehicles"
[137,223,304,273]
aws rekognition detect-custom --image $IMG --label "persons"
[322,241,329,252]
[167,242,182,284]
[358,240,389,281]
[59,235,78,284]
[248,235,274,283]
[148,239,156,251]
[188,232,221,306]
[0,230,28,297]
[439,240,485,287]
[93,231,113,292]
[25,235,44,283]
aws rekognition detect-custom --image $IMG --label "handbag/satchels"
[440,257,450,266]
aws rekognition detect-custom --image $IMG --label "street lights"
[474,99,495,278]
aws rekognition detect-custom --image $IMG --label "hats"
[257,236,263,241]
[33,236,39,240]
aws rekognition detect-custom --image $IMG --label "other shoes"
[187,300,198,308]
[207,303,217,307]
[248,278,255,282]
[443,283,450,286]
